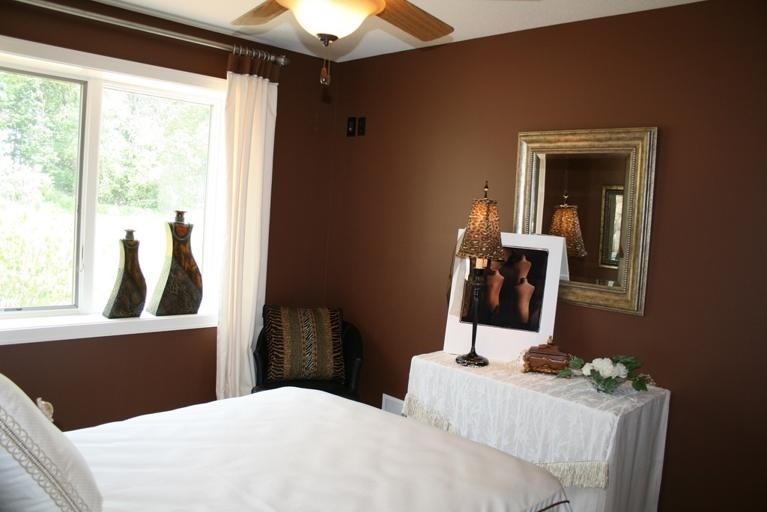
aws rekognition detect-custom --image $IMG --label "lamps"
[547,190,588,259]
[274,1,386,86]
[450,180,507,366]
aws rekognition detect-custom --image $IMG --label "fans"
[230,1,454,43]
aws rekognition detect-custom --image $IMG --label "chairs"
[251,322,364,399]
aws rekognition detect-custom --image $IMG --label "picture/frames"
[442,228,564,367]
[596,185,624,270]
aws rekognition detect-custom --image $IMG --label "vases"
[583,374,626,394]
[101,229,147,319]
[145,209,203,316]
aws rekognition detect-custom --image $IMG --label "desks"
[400,351,671,512]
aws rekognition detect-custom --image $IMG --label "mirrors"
[512,125,659,316]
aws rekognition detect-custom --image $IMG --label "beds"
[0,370,573,511]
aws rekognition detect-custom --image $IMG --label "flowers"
[551,351,650,394]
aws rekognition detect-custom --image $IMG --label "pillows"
[0,371,102,511]
[265,304,346,385]
